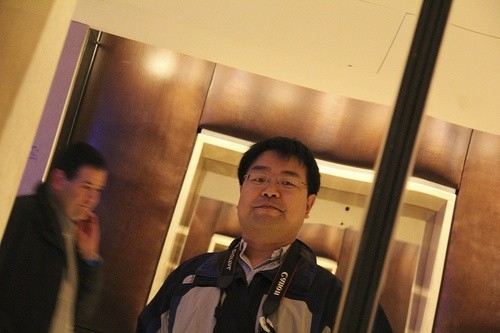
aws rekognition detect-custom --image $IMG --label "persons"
[1,142,108,333]
[136,135,344,333]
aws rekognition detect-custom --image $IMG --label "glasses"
[243,173,308,188]
